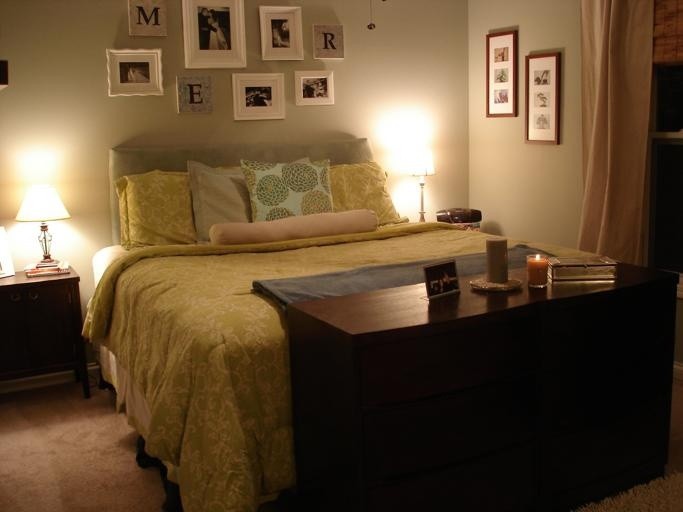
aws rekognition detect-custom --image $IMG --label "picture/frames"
[106,47,164,96]
[182,0,248,69]
[0,225,16,278]
[523,50,561,145]
[295,71,334,106]
[231,72,286,120]
[259,5,304,61]
[485,30,517,118]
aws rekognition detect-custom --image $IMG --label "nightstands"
[0,269,92,401]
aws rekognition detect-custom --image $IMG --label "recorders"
[437,208,481,223]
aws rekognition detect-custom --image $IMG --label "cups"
[485,290,510,312]
[527,285,549,303]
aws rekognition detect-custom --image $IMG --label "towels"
[252,242,556,313]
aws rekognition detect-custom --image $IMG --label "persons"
[198,8,217,51]
[207,10,228,51]
[246,89,272,107]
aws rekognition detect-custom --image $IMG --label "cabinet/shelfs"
[287,261,678,512]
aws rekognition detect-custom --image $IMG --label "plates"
[469,277,523,291]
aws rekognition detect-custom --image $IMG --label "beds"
[80,138,614,512]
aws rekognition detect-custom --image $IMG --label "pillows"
[240,155,332,223]
[207,208,379,243]
[326,162,400,224]
[110,158,198,247]
[185,157,312,240]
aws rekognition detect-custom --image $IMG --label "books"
[24,259,71,278]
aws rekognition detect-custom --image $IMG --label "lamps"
[399,143,435,222]
[12,184,71,268]
